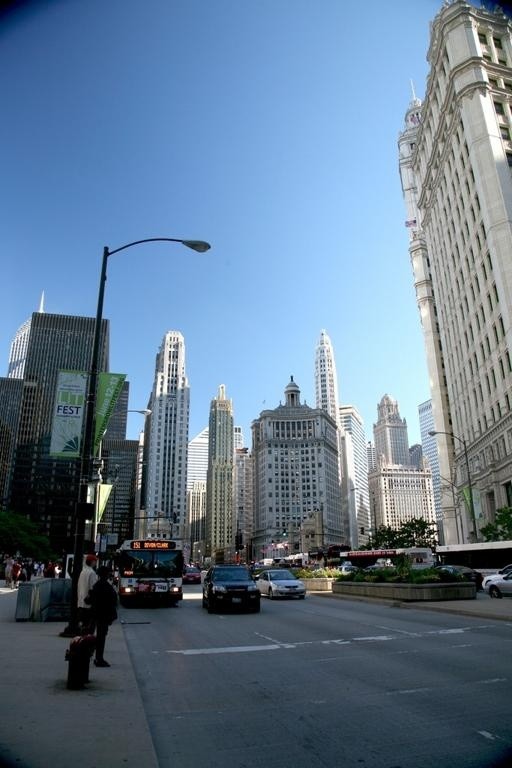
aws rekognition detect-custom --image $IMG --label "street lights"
[110,458,148,561]
[348,487,379,549]
[91,407,154,545]
[428,430,479,542]
[66,235,214,637]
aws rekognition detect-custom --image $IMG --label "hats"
[87,555,97,561]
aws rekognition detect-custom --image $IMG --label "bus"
[259,541,512,585]
[116,537,186,611]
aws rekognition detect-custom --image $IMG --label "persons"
[1,554,121,667]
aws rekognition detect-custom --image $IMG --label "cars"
[253,569,307,601]
[183,562,202,584]
[277,559,511,600]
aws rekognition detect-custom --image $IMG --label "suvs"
[202,563,261,614]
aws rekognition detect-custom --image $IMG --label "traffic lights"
[360,526,364,535]
[282,528,287,537]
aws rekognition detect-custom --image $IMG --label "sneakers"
[94,658,111,667]
